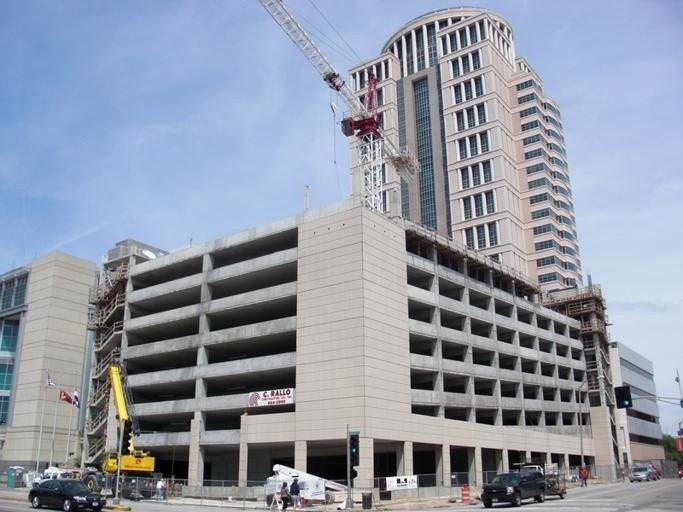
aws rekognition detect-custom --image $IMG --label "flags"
[60,389,72,404]
[74,390,80,407]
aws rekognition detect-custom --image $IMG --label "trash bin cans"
[362,493,372,509]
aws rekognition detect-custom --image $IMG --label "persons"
[579,467,588,487]
[155,478,164,501]
[279,482,290,512]
[290,478,300,512]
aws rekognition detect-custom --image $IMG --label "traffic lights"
[613,385,633,410]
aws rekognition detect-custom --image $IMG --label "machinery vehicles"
[85,363,157,500]
[263,464,347,506]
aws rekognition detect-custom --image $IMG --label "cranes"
[256,0,422,213]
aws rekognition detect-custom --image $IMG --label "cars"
[28,469,106,512]
[524,465,544,475]
[677,465,683,478]
[629,463,662,483]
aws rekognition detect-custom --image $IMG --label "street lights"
[577,380,587,487]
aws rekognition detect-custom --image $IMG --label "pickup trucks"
[481,471,548,508]
